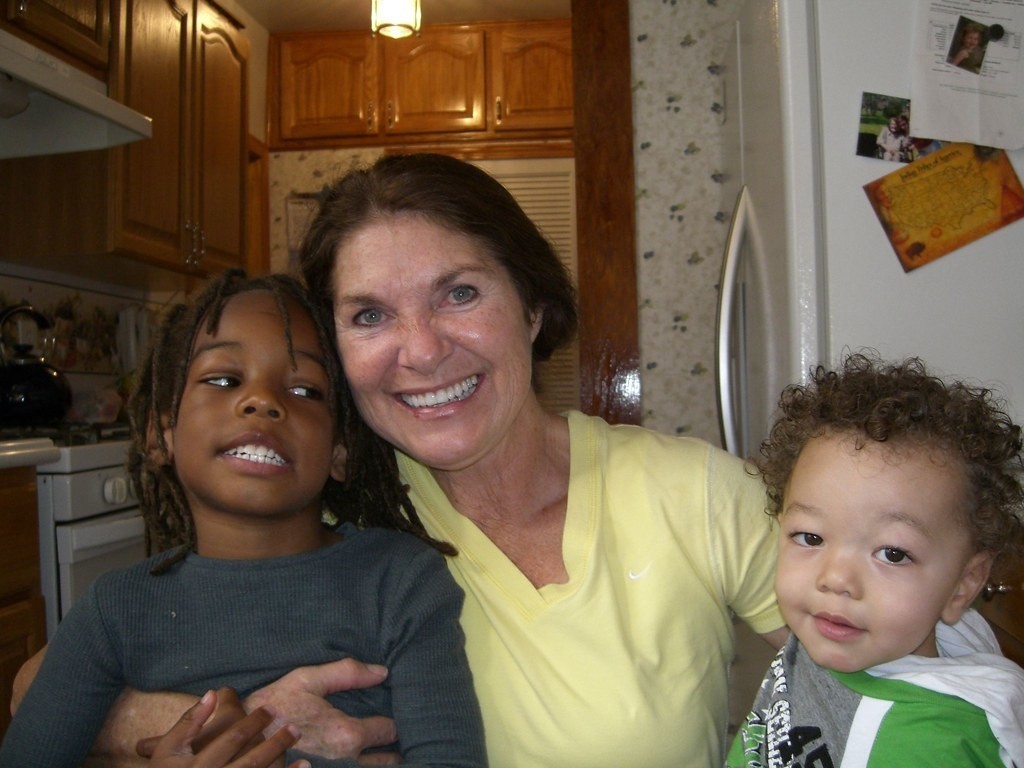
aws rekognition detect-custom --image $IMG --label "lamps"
[371,0,422,39]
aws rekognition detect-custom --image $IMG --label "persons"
[876,23,984,164]
[12,149,792,768]
[722,349,1024,768]
[0,266,489,768]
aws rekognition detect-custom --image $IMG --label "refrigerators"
[717,0,1024,525]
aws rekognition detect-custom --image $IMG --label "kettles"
[0,305,76,426]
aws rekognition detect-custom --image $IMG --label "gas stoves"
[0,421,140,521]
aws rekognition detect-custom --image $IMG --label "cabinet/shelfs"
[0,0,251,288]
[259,16,575,152]
[0,463,52,743]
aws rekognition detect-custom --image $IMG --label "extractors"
[0,27,152,158]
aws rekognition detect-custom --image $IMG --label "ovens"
[39,508,150,643]
